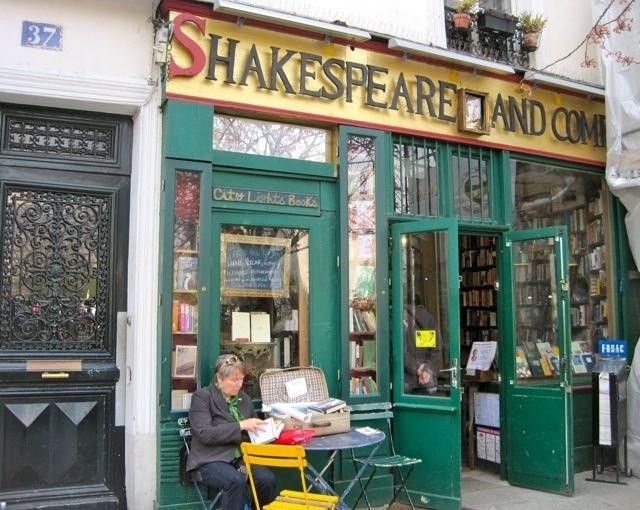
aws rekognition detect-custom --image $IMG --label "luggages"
[259,366,351,441]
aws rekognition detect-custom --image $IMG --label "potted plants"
[452,0,479,27]
[516,9,548,46]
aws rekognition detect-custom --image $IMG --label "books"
[232,311,270,343]
[459,235,498,347]
[514,196,609,378]
[170,257,198,410]
[309,398,346,414]
[349,234,377,394]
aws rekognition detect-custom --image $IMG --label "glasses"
[219,354,245,370]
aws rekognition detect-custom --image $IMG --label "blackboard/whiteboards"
[219,233,291,299]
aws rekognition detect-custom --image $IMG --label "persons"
[465,92,485,131]
[188,354,270,509]
[402,295,441,396]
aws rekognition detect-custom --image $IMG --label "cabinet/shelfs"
[458,202,609,369]
[171,248,198,335]
[348,193,378,377]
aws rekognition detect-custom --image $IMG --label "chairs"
[178,401,423,510]
